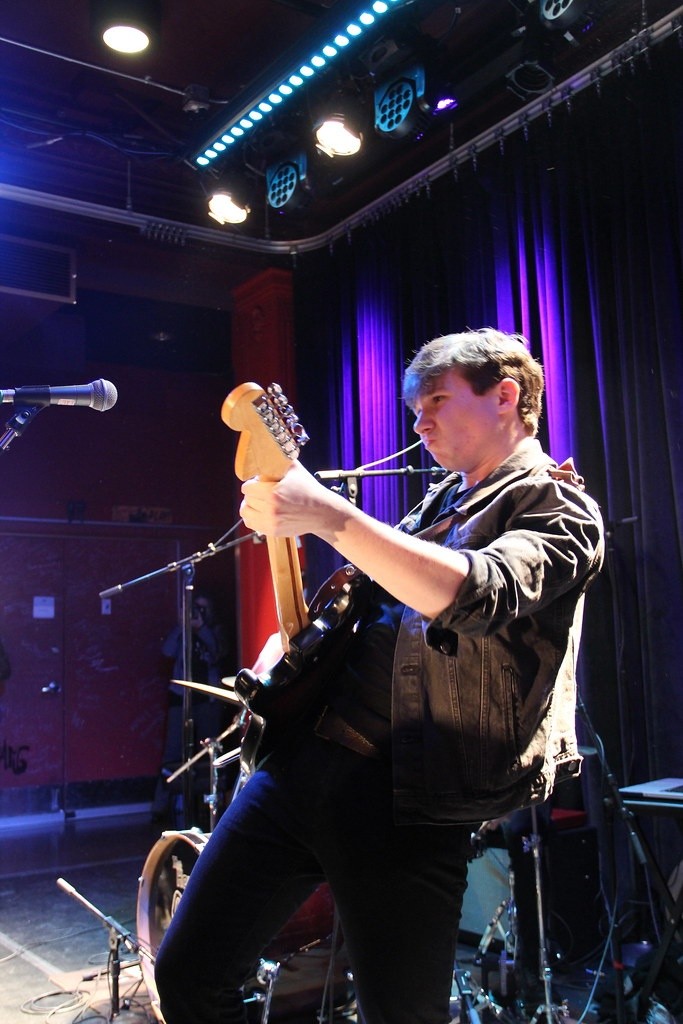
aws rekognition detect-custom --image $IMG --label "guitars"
[218,379,364,773]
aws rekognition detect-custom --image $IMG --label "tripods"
[529,809,572,1024]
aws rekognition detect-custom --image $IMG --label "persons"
[150,591,221,826]
[154,328,606,1024]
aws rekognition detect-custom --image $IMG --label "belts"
[297,697,376,756]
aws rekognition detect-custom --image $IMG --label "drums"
[137,824,347,1023]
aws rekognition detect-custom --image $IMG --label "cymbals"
[167,678,240,706]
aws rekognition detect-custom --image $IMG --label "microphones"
[473,904,506,963]
[0,379,118,411]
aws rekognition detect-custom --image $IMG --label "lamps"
[207,157,259,224]
[313,87,367,158]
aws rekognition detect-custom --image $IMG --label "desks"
[601,776,683,1024]
